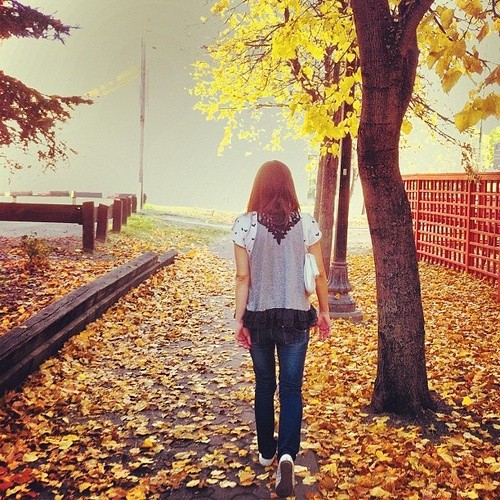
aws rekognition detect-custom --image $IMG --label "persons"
[230,160,332,498]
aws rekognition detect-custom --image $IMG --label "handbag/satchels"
[302,212,319,294]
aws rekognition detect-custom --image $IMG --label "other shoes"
[275,454,296,496]
[259,453,276,466]
